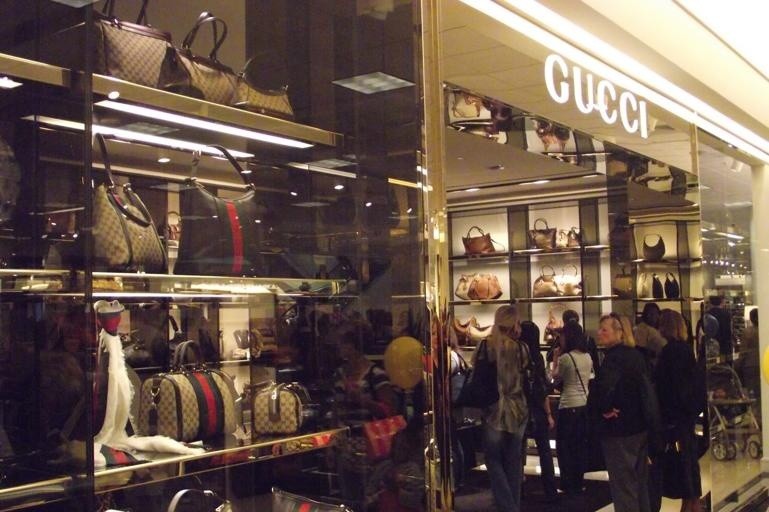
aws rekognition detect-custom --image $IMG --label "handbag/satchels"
[534,264,582,297]
[138,340,237,443]
[89,183,168,273]
[527,405,548,438]
[544,305,568,342]
[173,180,268,279]
[613,275,635,298]
[450,360,498,410]
[230,55,295,121]
[462,226,496,255]
[526,218,580,248]
[90,19,176,92]
[453,318,494,345]
[367,414,411,462]
[455,274,503,300]
[168,488,238,511]
[272,487,352,512]
[644,234,665,261]
[664,272,679,297]
[164,48,236,105]
[663,448,703,499]
[636,274,663,298]
[252,384,303,435]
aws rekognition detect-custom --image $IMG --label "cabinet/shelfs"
[448,198,704,456]
[0,52,433,512]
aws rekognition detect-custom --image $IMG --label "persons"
[450,296,757,512]
[276,303,427,512]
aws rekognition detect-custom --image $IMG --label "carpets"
[460,469,613,512]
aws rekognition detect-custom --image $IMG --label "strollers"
[707,364,762,461]
